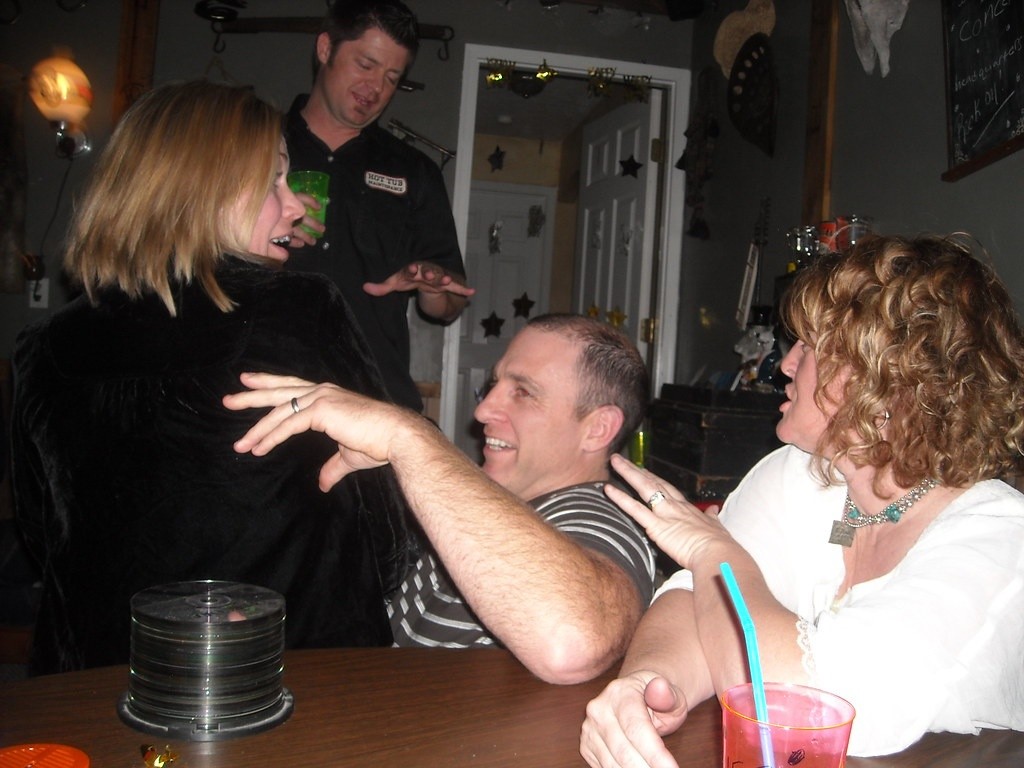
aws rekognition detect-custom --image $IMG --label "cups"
[788,225,818,268]
[287,171,329,236]
[628,428,651,467]
[722,682,855,768]
[834,214,873,252]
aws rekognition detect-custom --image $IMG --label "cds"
[128,579,287,730]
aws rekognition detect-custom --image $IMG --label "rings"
[291,398,299,413]
[648,491,665,508]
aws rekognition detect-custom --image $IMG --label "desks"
[646,382,794,505]
[0,640,1024,768]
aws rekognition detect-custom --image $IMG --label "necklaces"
[829,478,935,548]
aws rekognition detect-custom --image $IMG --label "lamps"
[24,57,98,160]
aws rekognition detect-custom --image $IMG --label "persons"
[578,237,1024,767]
[0,83,393,675]
[282,0,475,412]
[223,313,656,686]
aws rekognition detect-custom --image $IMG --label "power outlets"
[28,277,50,310]
